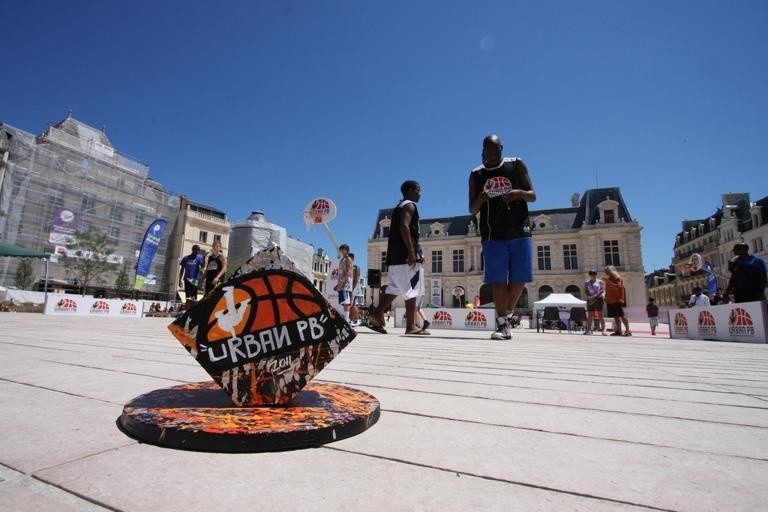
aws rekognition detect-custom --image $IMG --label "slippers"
[365,322,387,335]
[403,327,432,337]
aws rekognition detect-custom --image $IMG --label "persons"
[581,268,608,337]
[199,240,227,294]
[599,264,632,338]
[701,285,735,307]
[464,301,473,310]
[330,244,354,323]
[362,179,434,337]
[175,244,209,301]
[645,296,660,337]
[348,252,362,327]
[414,267,430,331]
[722,243,768,304]
[688,287,711,308]
[467,133,537,341]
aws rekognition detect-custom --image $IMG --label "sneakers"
[422,321,431,329]
[487,315,513,341]
[582,328,633,336]
[349,319,362,328]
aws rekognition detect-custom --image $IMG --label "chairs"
[541,307,561,333]
[567,307,588,333]
